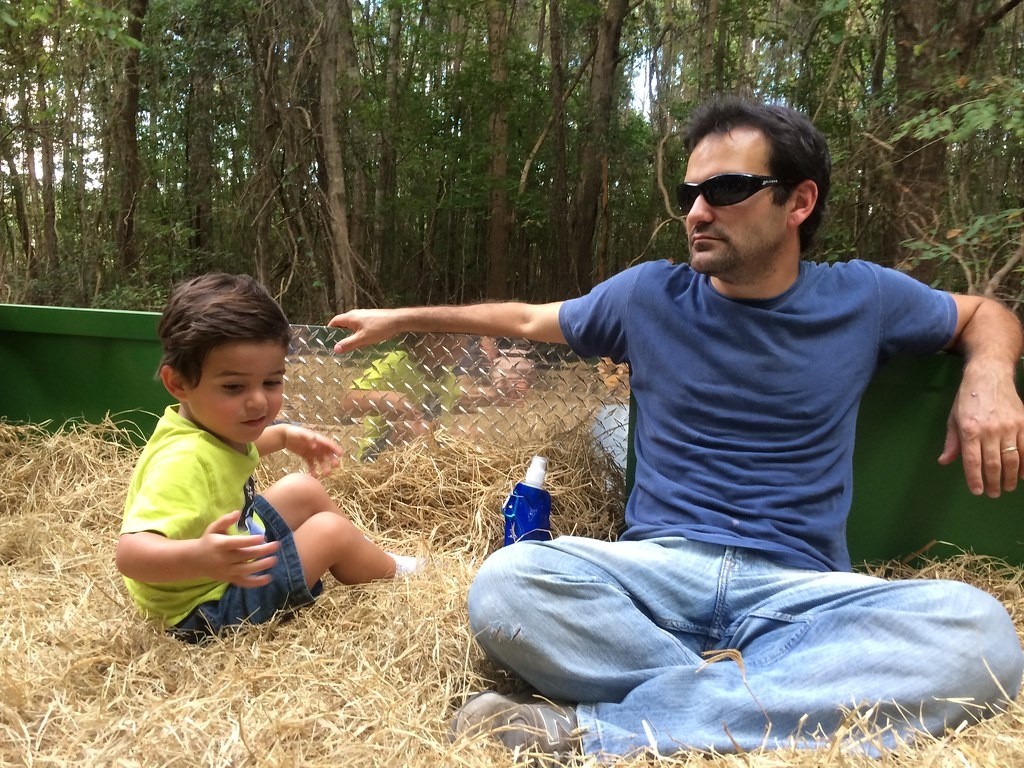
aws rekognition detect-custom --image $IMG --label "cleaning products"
[500,454,552,548]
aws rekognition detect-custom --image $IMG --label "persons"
[116,272,427,647]
[338,332,630,492]
[328,88,1024,768]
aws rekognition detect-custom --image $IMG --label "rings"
[1001,446,1021,454]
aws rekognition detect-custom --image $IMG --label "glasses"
[675,174,778,213]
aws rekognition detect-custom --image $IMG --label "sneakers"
[448,688,585,768]
